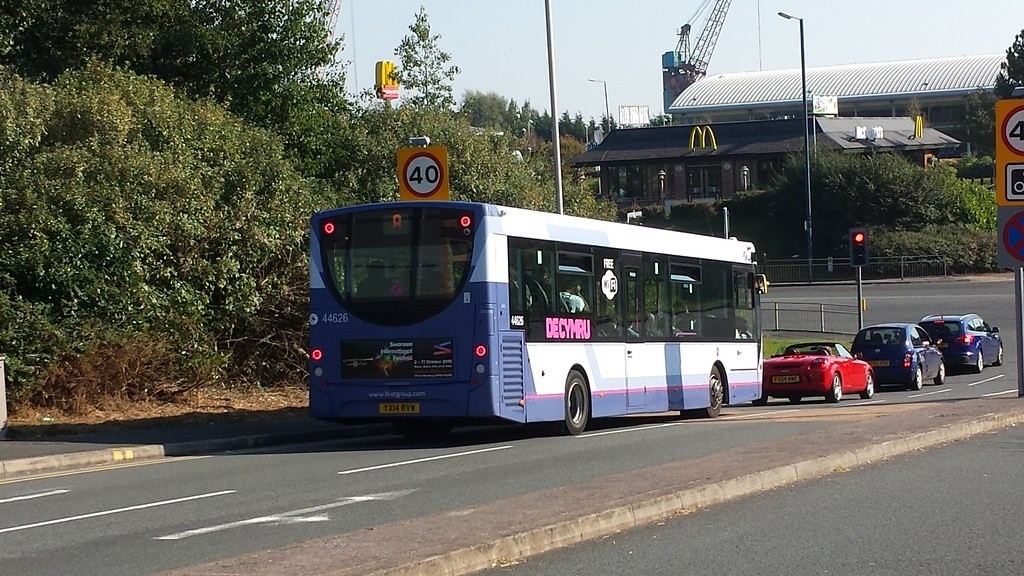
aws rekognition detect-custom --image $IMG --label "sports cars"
[752,342,875,406]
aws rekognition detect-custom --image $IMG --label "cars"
[850,322,946,392]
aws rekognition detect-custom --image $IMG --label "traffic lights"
[849,227,870,268]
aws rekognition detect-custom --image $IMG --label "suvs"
[917,313,1004,376]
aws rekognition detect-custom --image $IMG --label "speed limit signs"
[397,146,449,200]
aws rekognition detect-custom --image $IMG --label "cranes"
[662,0,731,114]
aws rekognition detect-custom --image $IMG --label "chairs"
[510,279,519,309]
[874,333,885,344]
[527,278,549,311]
[817,349,829,356]
[785,348,797,354]
[939,326,952,336]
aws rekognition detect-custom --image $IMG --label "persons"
[509,266,533,310]
[538,264,553,301]
[564,278,590,313]
[356,259,395,297]
[672,301,694,334]
[795,348,801,354]
[822,346,834,356]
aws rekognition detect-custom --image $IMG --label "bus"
[307,201,768,440]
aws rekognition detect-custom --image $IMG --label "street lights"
[588,79,610,133]
[778,12,814,283]
